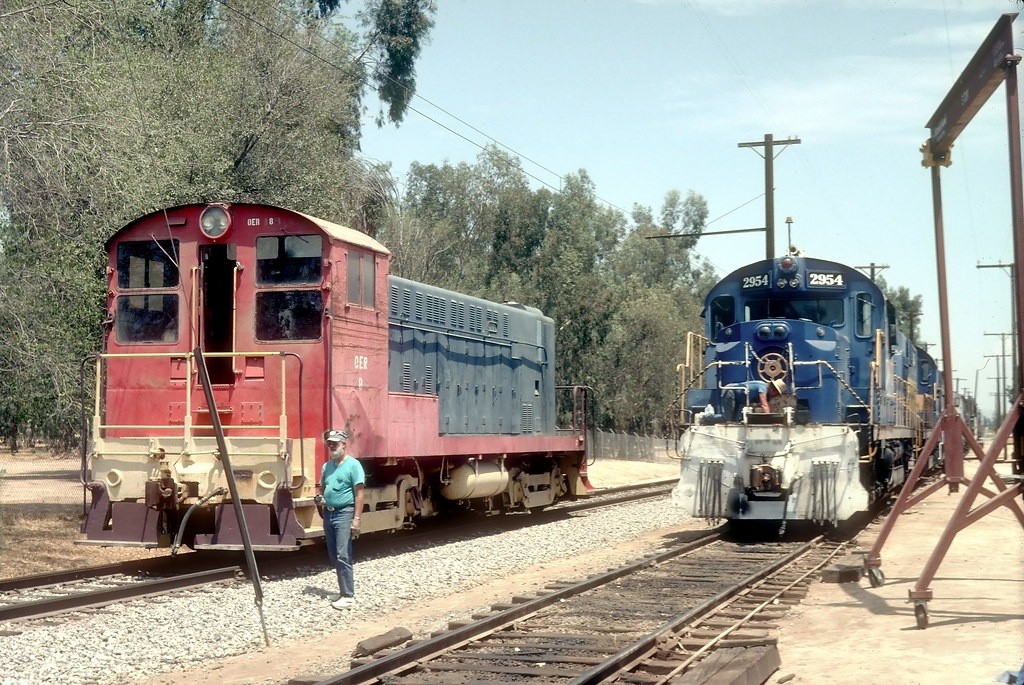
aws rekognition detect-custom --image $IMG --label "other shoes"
[694,412,698,423]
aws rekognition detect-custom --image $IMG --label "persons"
[314,429,366,609]
[699,379,786,424]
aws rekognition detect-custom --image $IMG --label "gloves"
[314,494,326,506]
[351,518,362,535]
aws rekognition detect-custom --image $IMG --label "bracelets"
[354,517,360,520]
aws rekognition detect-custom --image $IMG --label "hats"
[770,377,786,394]
[324,430,348,442]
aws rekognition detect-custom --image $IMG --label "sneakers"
[331,596,355,609]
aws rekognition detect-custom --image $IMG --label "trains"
[73,201,610,558]
[664,218,985,538]
